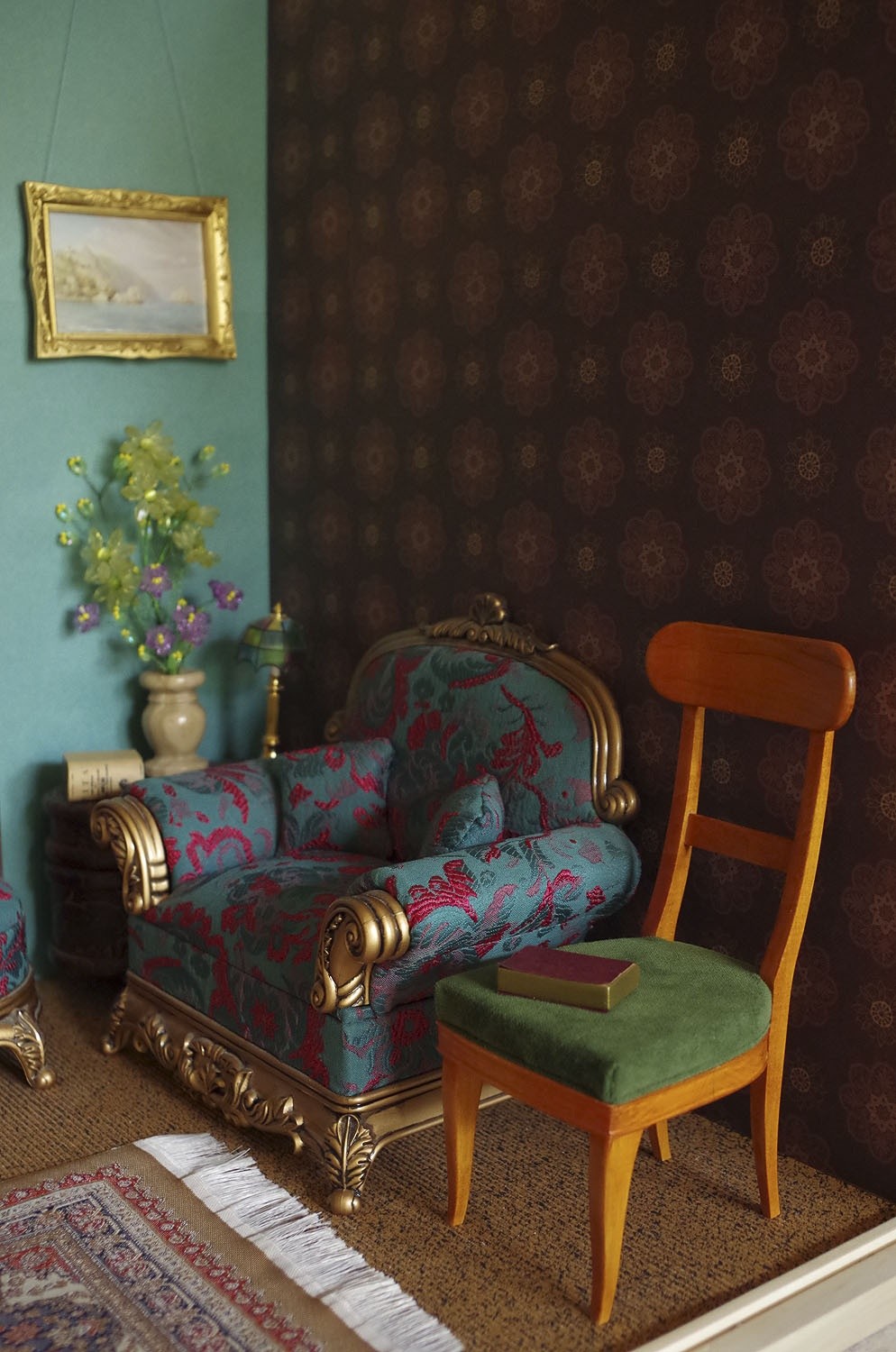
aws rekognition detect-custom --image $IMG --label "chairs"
[434,622,858,1325]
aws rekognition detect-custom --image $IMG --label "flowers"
[58,417,242,677]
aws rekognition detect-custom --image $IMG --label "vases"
[137,666,214,777]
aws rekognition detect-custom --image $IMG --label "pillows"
[417,773,506,859]
[268,737,394,857]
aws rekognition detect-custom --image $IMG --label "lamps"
[236,602,311,757]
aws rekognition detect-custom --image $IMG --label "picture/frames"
[23,181,238,363]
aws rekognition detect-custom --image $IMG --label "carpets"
[0,1133,462,1352]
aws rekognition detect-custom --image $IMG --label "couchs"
[91,592,643,1213]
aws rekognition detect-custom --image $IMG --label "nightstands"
[41,792,129,977]
